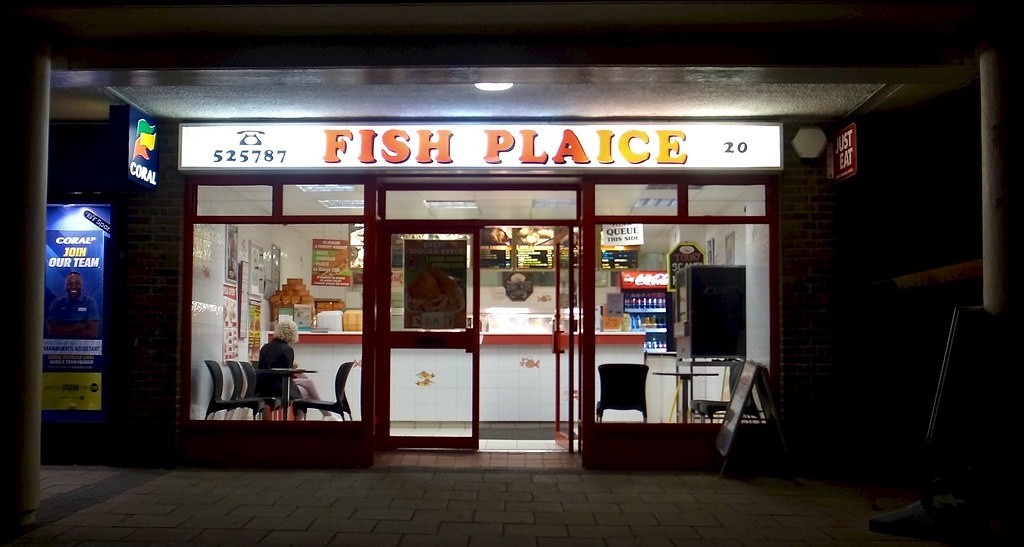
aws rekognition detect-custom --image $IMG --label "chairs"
[224,360,281,420]
[204,360,264,420]
[239,362,291,421]
[293,361,355,421]
[690,362,762,424]
[596,363,649,422]
[251,361,294,421]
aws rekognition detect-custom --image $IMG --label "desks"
[653,372,720,423]
[258,369,318,420]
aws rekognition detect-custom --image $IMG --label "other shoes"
[322,412,344,421]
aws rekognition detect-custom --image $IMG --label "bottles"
[631,314,666,329]
[643,333,667,348]
[624,291,666,309]
[603,310,631,332]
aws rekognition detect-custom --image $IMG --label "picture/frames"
[725,230,735,265]
[706,238,714,265]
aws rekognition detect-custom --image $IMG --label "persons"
[48,272,99,340]
[250,320,344,421]
